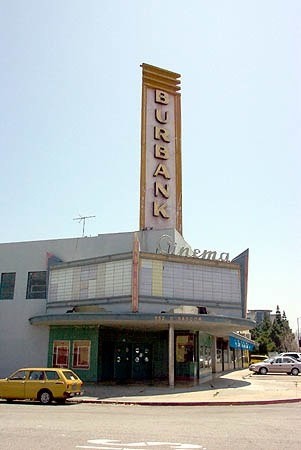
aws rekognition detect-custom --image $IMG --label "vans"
[250,355,268,363]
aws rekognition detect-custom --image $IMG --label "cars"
[279,351,301,363]
[248,356,301,374]
[0,367,83,405]
